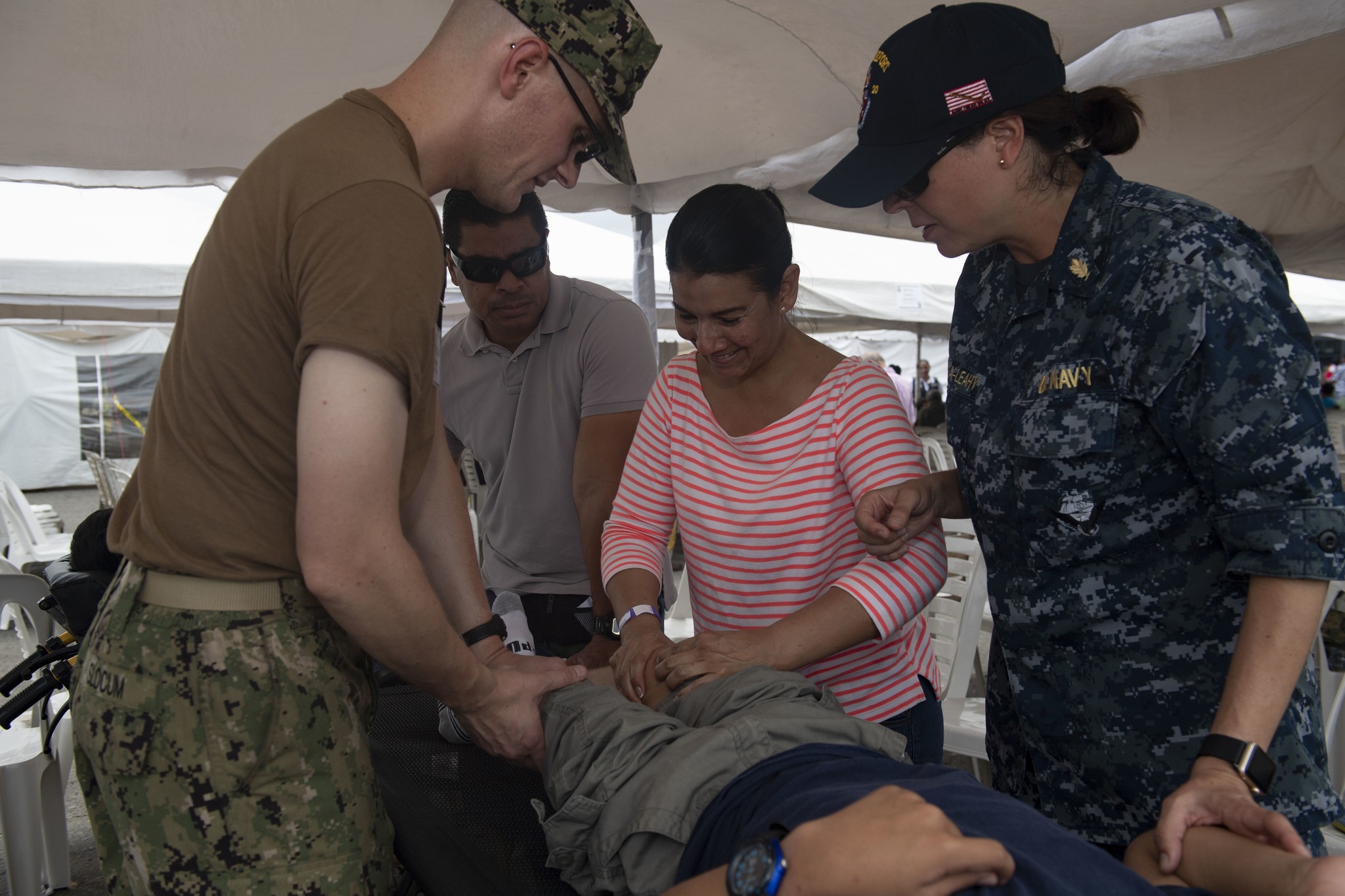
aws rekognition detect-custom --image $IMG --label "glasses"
[893,117,991,200]
[445,232,548,282]
[510,43,608,163]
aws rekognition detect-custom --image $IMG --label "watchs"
[725,839,789,896]
[594,613,621,641]
[1196,729,1278,795]
[458,612,508,647]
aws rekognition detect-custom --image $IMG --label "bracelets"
[619,604,662,632]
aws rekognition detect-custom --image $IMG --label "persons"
[73,0,661,896]
[861,351,917,428]
[438,591,1345,896]
[808,1,1345,857]
[433,186,679,669]
[1320,353,1345,403]
[912,359,945,428]
[602,183,949,766]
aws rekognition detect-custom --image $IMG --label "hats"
[494,0,663,186]
[808,3,1065,208]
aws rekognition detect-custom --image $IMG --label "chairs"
[0,449,131,896]
[663,424,1344,799]
